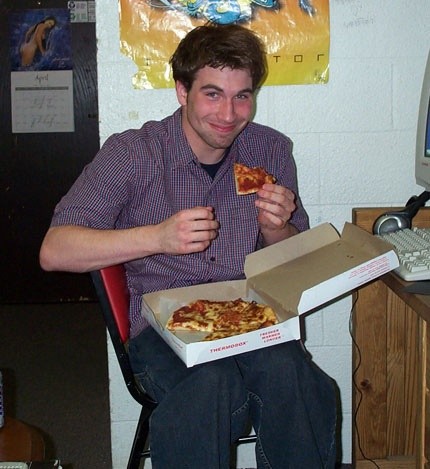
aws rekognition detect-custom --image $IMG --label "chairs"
[88,263,344,469]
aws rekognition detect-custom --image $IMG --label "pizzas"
[233,162,277,195]
[167,297,279,341]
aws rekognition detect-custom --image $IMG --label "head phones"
[373,191,430,233]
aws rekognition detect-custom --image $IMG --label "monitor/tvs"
[415,41,430,188]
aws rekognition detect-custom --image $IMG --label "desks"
[350,205,430,469]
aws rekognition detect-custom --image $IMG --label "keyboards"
[375,226,430,283]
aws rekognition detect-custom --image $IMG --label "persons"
[19,17,56,66]
[38,24,343,469]
